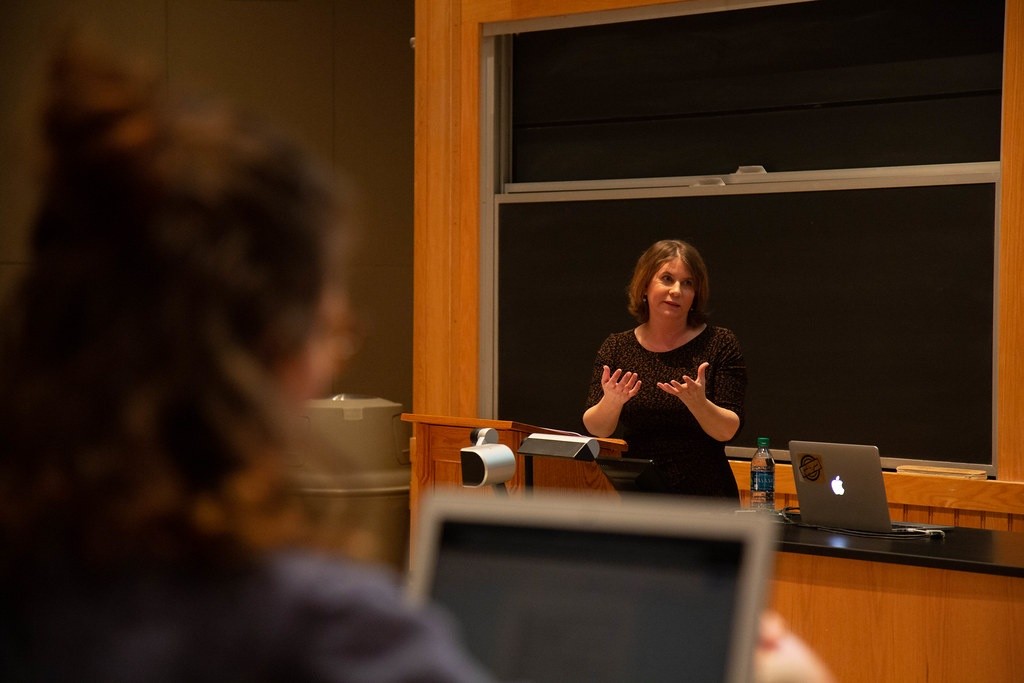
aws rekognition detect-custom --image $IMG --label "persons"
[0,38,482,683]
[582,241,749,500]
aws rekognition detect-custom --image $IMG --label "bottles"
[750,438,775,511]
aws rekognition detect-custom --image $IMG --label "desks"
[762,510,1024,683]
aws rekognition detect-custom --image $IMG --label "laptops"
[410,487,785,683]
[788,440,955,533]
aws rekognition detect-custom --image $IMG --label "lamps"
[516,432,600,493]
